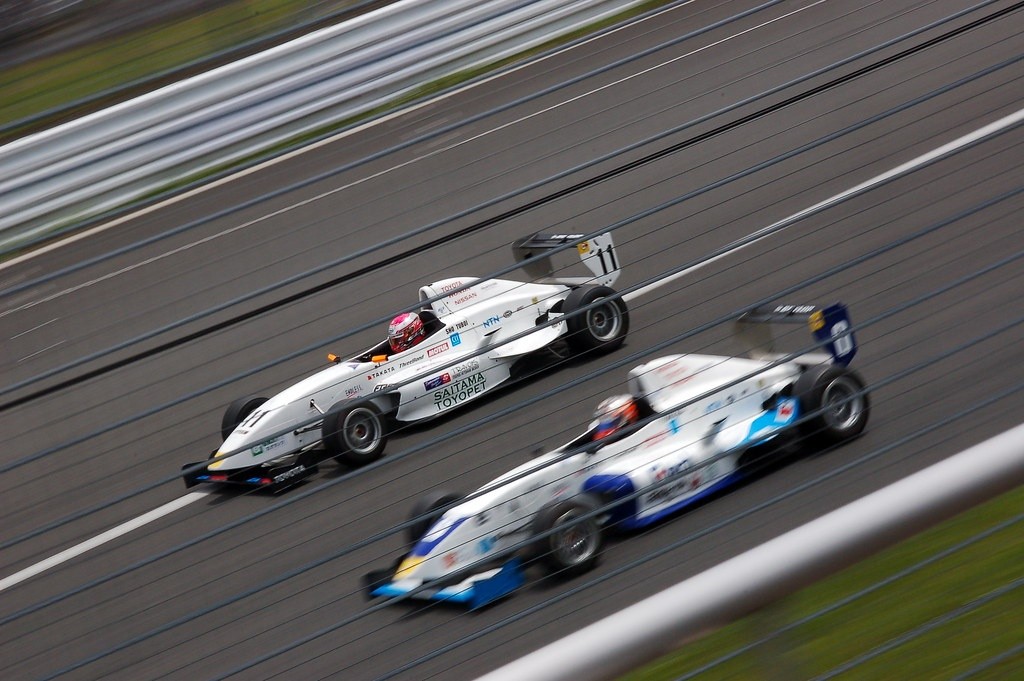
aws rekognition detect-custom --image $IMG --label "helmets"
[593,393,638,441]
[387,312,425,353]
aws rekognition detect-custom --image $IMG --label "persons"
[387,312,427,354]
[589,394,638,441]
